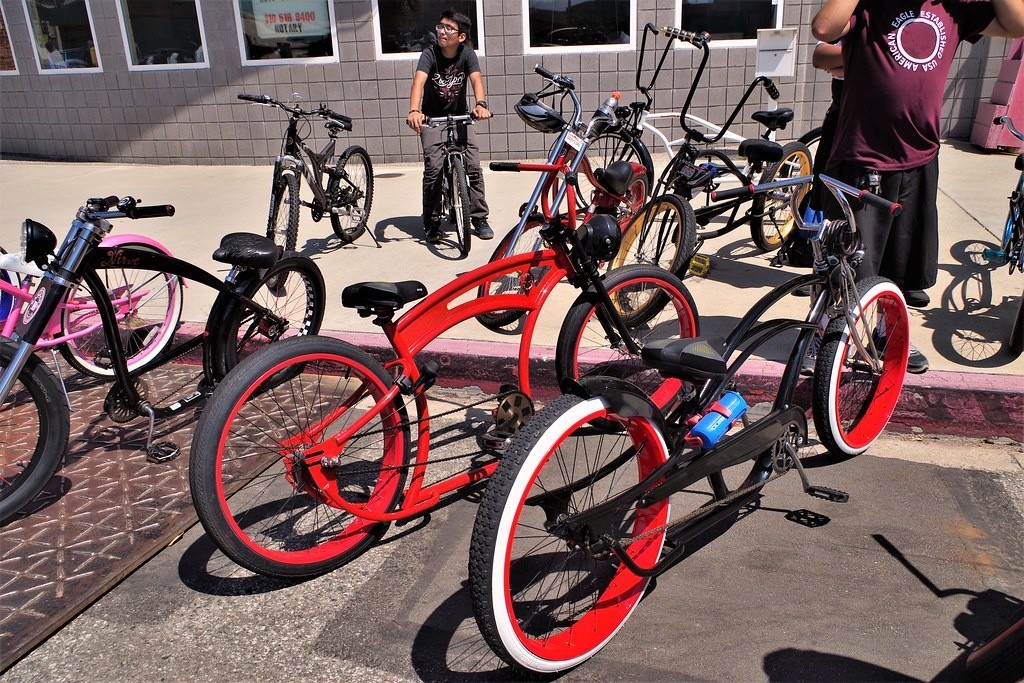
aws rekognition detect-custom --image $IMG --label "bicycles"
[991,115,1024,361]
[406,112,493,254]
[469,177,913,682]
[190,162,702,581]
[233,88,377,291]
[0,195,326,522]
[476,22,816,326]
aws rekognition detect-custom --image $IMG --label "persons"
[779,0,1024,373]
[407,12,494,241]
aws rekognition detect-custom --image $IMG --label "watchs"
[476,101,487,108]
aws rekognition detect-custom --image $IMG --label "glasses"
[436,23,460,34]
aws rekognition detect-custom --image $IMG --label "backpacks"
[771,192,826,268]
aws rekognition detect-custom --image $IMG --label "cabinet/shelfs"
[969,37,1024,155]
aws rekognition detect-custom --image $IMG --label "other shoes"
[801,331,821,374]
[425,226,443,241]
[903,289,930,307]
[875,337,929,374]
[791,284,817,296]
[470,216,494,240]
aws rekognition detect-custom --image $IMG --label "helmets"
[514,94,566,134]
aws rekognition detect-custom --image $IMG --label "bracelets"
[409,110,420,114]
